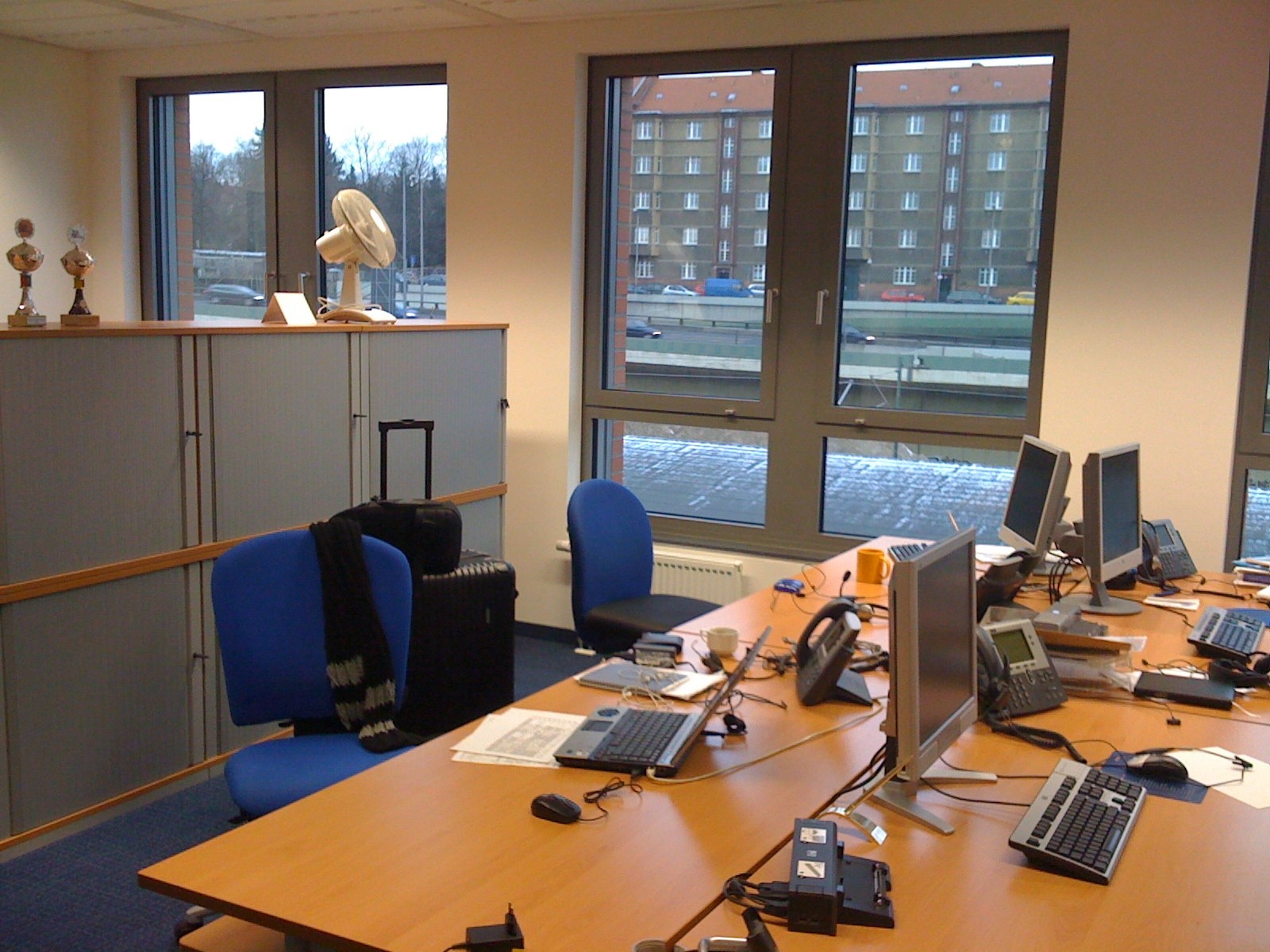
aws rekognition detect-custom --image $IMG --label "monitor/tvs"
[1059,442,1143,615]
[862,527,998,835]
[997,432,1074,575]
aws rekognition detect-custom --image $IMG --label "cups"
[856,549,890,584]
[699,628,738,655]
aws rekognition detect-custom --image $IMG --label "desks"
[135,533,1269,951]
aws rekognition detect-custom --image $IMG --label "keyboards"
[1008,758,1148,886]
[1188,606,1265,664]
[887,542,929,563]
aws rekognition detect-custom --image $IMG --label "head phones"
[1208,650,1270,690]
[856,603,890,622]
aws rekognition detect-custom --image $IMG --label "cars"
[1006,291,1036,305]
[203,284,265,307]
[947,289,1002,304]
[880,288,926,302]
[394,304,422,319]
[626,317,663,339]
[626,277,765,299]
[397,271,445,286]
[841,322,875,345]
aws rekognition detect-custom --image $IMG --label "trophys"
[61,223,100,326]
[6,218,47,327]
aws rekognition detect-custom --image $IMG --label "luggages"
[377,416,520,745]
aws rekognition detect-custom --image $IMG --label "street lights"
[632,208,640,295]
[401,164,413,320]
[408,171,435,307]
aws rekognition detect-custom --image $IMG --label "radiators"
[556,540,740,654]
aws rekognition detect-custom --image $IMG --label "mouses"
[531,793,581,824]
[1126,753,1188,783]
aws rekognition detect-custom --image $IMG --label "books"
[1133,670,1235,710]
[578,661,689,699]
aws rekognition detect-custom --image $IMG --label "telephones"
[973,617,1070,722]
[795,597,862,704]
[1139,518,1198,583]
[976,549,1041,622]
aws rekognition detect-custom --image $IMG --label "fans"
[316,187,397,325]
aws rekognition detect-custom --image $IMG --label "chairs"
[567,479,722,655]
[172,530,436,943]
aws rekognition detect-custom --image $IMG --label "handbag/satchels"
[339,494,461,575]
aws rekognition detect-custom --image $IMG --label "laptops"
[552,625,773,779]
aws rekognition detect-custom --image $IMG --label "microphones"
[840,570,852,597]
